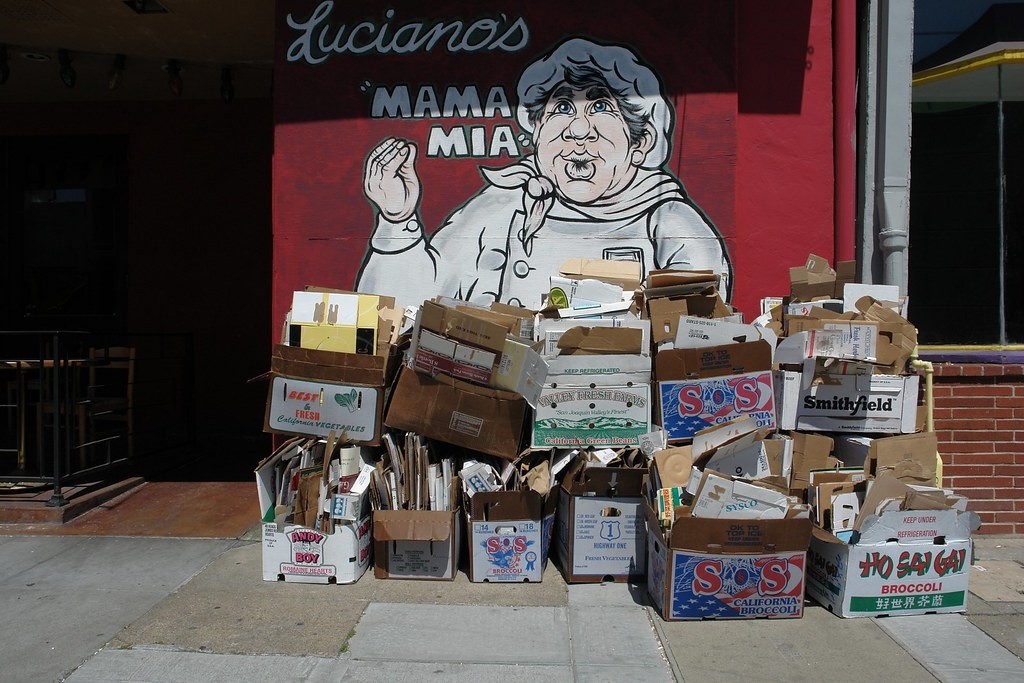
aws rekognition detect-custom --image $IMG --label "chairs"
[37,346,136,471]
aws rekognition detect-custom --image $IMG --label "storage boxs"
[253,253,982,624]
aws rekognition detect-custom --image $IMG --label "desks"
[0,359,110,470]
